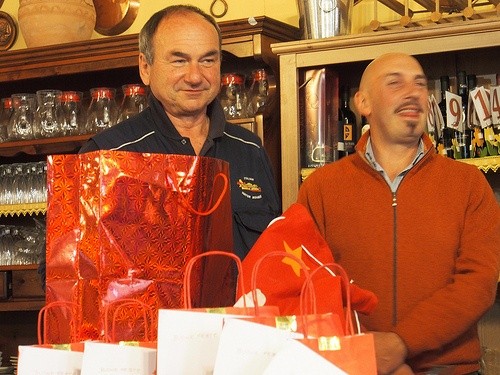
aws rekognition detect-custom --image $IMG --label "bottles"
[0,69,272,146]
[458,71,471,160]
[436,74,457,160]
[465,73,478,96]
[0,159,49,268]
[338,81,355,161]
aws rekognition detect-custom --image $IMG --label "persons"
[39,5,283,302]
[294,51,500,375]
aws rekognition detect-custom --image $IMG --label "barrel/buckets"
[294,0,355,43]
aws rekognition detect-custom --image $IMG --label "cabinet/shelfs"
[1,0,500,374]
